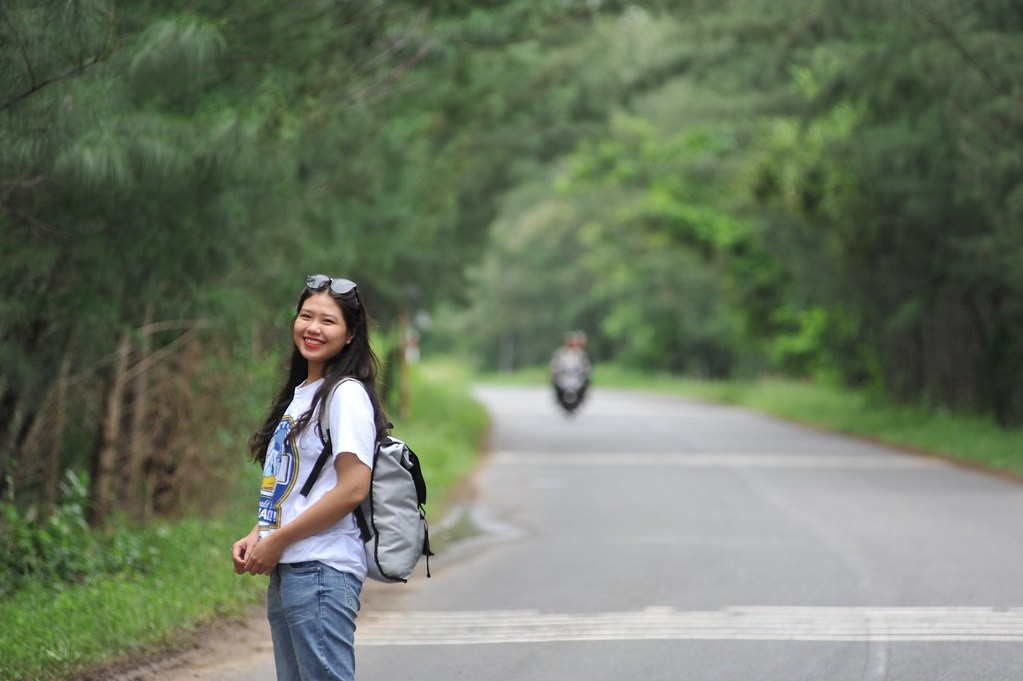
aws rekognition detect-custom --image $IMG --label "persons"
[230,271,392,681]
[551,331,590,383]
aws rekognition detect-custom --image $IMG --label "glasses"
[306,273,358,295]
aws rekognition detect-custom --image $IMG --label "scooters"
[551,350,592,413]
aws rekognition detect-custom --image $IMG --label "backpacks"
[298,376,435,585]
